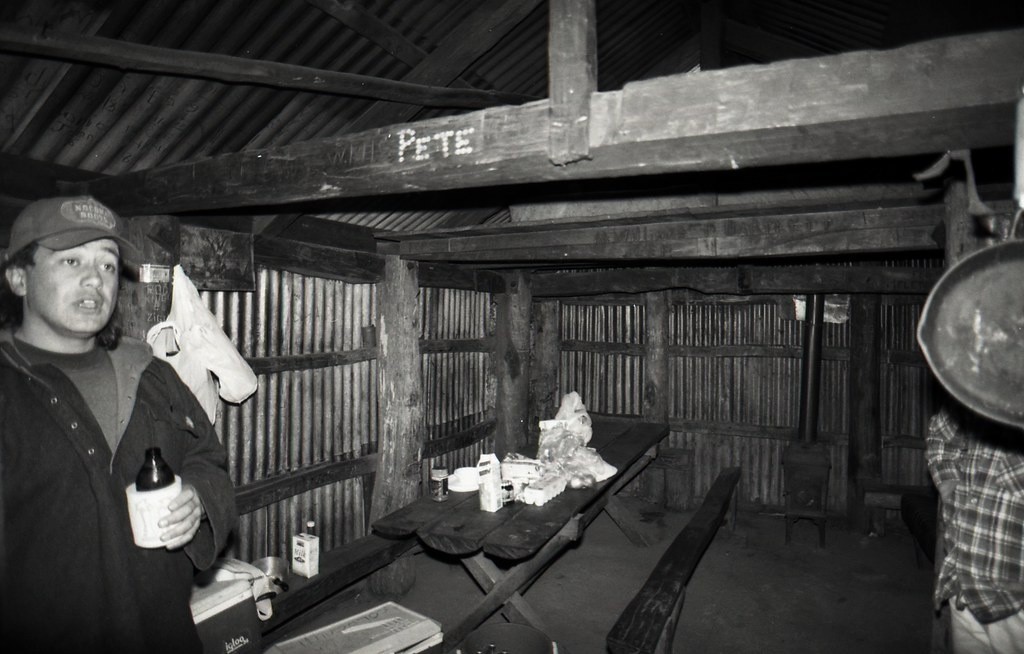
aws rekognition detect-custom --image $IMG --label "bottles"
[501,480,514,507]
[430,466,452,502]
[306,520,316,536]
[135,447,176,491]
[476,641,509,654]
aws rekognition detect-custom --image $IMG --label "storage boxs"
[267,602,445,653]
[189,566,264,654]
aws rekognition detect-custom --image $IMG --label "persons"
[926,408,1024,654]
[0,193,235,654]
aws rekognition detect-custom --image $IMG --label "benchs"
[252,532,420,637]
[605,465,743,654]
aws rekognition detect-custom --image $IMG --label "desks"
[371,420,671,653]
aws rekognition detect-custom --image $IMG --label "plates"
[447,474,480,492]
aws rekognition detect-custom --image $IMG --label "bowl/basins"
[454,467,476,484]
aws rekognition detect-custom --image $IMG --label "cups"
[125,473,183,549]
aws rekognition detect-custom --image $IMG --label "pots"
[915,240,1024,431]
[250,556,291,595]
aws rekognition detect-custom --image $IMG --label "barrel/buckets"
[456,622,560,654]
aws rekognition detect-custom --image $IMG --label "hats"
[5,194,136,263]
